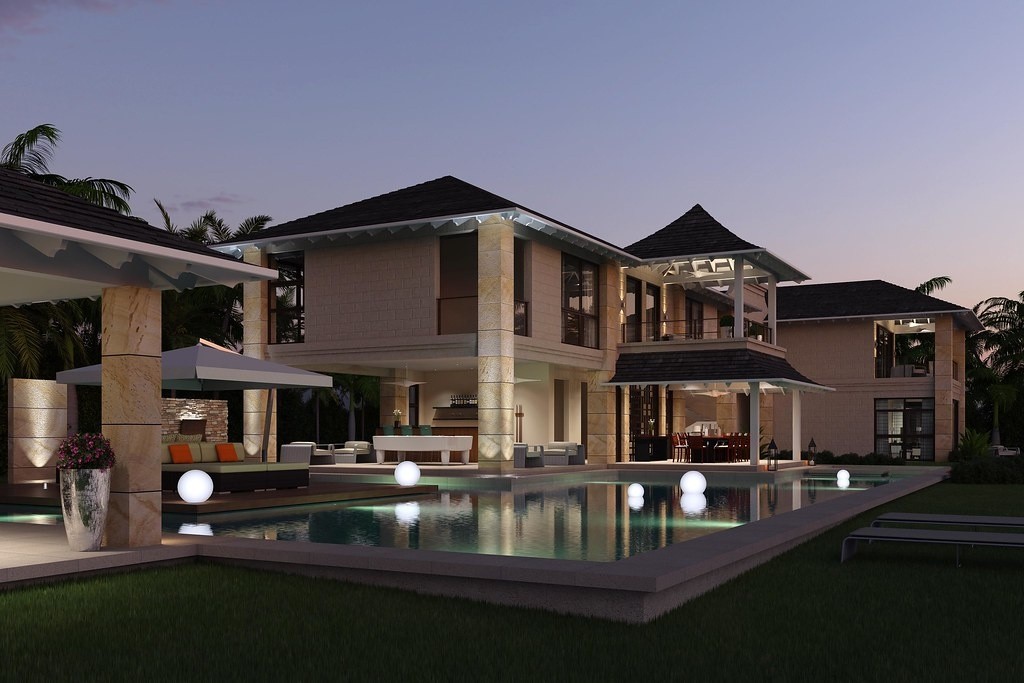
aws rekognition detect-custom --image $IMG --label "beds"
[160,443,310,494]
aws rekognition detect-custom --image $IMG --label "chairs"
[400,424,414,461]
[420,424,440,462]
[671,431,750,463]
[381,423,398,462]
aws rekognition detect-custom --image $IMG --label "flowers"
[55,432,117,469]
[393,409,402,420]
[648,419,655,425]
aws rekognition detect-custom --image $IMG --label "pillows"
[168,444,193,464]
[176,433,203,443]
[161,434,176,443]
[214,443,239,462]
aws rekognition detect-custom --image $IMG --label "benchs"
[840,512,1024,564]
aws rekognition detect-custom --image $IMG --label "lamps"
[837,470,850,480]
[394,461,421,486]
[680,494,707,516]
[837,480,850,489]
[680,470,707,494]
[627,483,644,497]
[628,497,644,511]
[176,470,214,502]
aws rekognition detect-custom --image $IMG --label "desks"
[635,435,668,462]
[372,435,473,464]
[528,444,544,451]
[703,436,729,464]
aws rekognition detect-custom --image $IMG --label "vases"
[59,469,112,552]
[394,421,401,428]
[648,425,653,436]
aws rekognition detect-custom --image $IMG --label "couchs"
[538,442,585,466]
[331,441,377,463]
[514,443,545,468]
[280,441,336,465]
